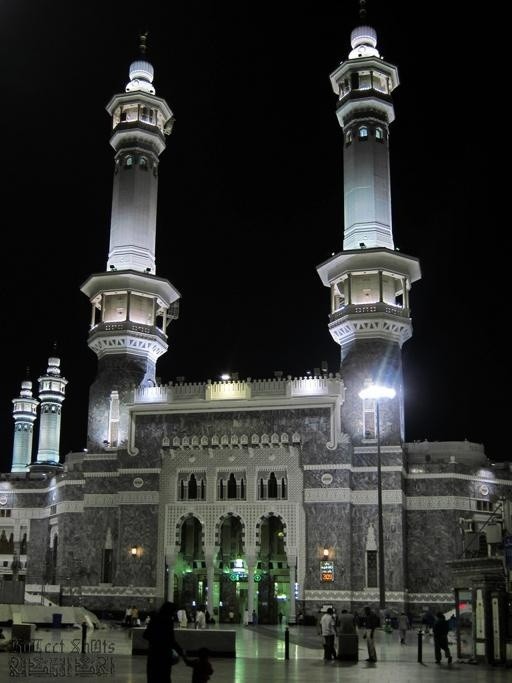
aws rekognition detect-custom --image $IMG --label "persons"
[251,609,257,625]
[279,612,283,625]
[230,609,234,624]
[243,608,249,627]
[316,606,377,663]
[125,600,214,683]
[434,611,452,664]
[421,611,436,630]
[396,610,415,645]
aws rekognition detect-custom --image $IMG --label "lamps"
[130,544,137,558]
[322,544,329,561]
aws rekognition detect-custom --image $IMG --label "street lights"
[359,383,396,627]
